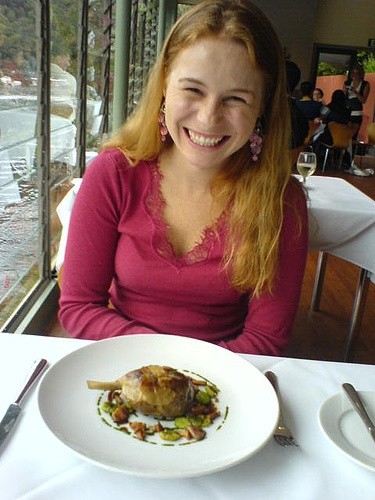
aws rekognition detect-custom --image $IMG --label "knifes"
[0,359,48,444]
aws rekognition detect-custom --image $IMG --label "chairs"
[318,122,359,173]
[353,115,369,154]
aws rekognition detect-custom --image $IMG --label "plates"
[38,334,280,478]
[319,391,375,471]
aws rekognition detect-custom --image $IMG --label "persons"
[57,0,309,357]
[296,64,371,171]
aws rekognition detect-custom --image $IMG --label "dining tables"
[0,333,375,500]
[55,173,375,362]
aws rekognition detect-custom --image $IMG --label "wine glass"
[296,152,317,190]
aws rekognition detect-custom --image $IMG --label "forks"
[264,371,298,448]
